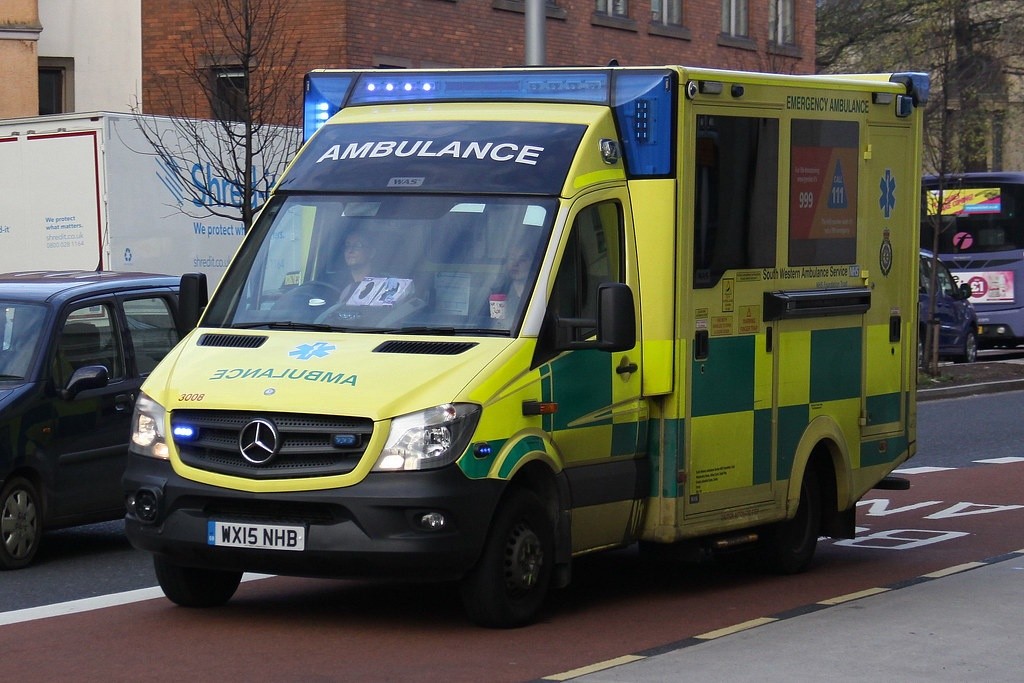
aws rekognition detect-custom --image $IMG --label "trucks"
[0,110,304,303]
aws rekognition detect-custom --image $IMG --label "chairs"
[62,323,102,370]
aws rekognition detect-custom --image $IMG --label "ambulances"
[124,68,933,628]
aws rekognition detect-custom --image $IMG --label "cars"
[0,270,181,570]
[917,247,979,364]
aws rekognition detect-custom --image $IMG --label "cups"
[488,294,508,319]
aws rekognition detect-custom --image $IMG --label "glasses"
[342,241,368,252]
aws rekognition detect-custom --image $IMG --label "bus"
[921,171,1024,348]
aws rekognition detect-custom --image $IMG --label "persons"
[483,242,559,361]
[324,231,389,311]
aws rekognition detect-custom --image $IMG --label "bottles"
[999,275,1006,297]
